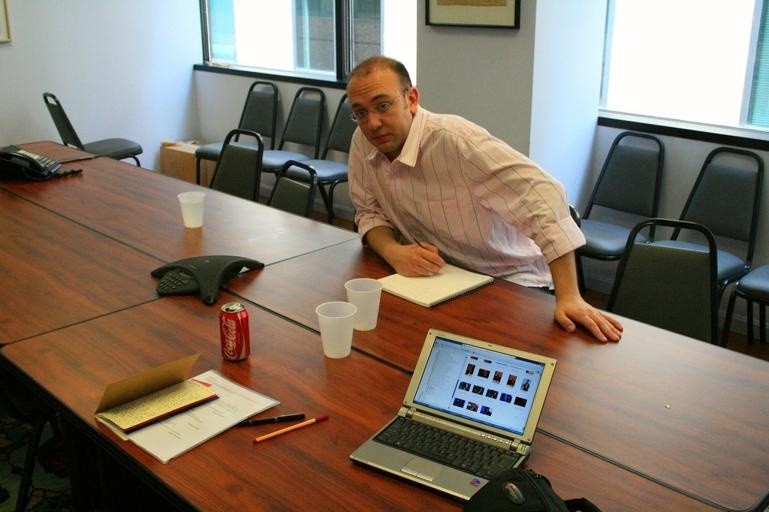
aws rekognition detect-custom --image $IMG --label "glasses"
[348,94,402,125]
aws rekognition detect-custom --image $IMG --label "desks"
[222,239,768,511]
[0,186,196,346]
[0,139,359,280]
[1,282,733,511]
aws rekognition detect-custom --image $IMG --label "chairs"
[193,81,359,224]
[42,92,143,167]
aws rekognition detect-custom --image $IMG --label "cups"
[343,277,380,331]
[176,191,207,229]
[317,301,358,359]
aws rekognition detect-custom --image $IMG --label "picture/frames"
[0,0,11,44]
[425,0,521,30]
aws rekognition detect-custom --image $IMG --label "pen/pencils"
[409,232,427,249]
[237,413,305,426]
[255,414,330,443]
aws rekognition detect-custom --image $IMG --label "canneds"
[219,303,250,362]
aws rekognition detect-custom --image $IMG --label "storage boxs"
[159,139,217,186]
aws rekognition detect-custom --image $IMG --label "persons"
[347,56,623,344]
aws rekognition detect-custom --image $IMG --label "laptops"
[349,329,557,501]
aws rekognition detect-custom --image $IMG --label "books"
[95,351,219,442]
[378,263,496,308]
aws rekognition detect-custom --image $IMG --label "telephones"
[151,255,264,305]
[0,145,62,181]
